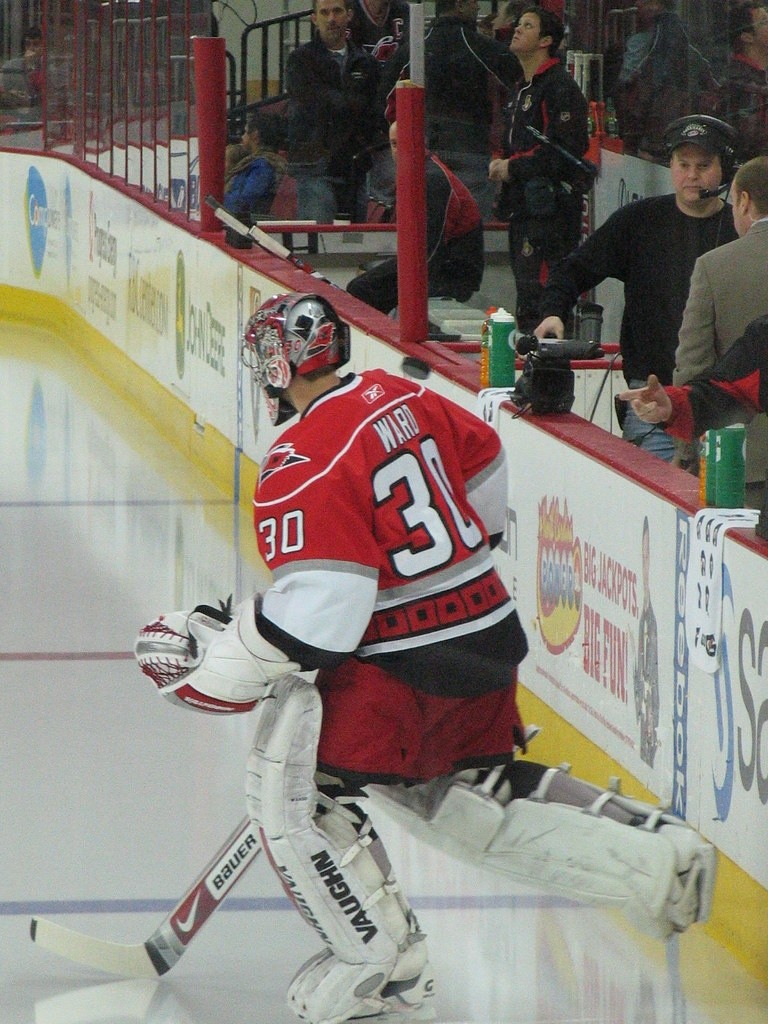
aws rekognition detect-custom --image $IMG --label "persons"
[133,294,715,1024]
[224,112,283,219]
[345,0,409,66]
[487,9,588,343]
[24,27,42,88]
[535,116,744,467]
[618,312,768,443]
[665,157,768,491]
[285,0,385,225]
[346,122,484,315]
[606,0,768,170]
[385,0,501,154]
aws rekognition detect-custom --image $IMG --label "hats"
[663,115,738,163]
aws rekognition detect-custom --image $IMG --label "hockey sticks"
[30,814,263,980]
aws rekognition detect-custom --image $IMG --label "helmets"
[245,292,351,426]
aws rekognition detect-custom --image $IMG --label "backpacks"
[268,173,298,221]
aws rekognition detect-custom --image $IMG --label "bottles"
[587,97,620,140]
[479,306,516,388]
[699,421,748,508]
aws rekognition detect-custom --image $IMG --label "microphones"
[517,337,532,355]
[699,181,732,201]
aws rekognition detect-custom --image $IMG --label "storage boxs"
[429,297,456,302]
[428,301,469,309]
[440,319,486,341]
[428,308,488,328]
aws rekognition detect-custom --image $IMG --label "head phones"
[662,114,739,182]
